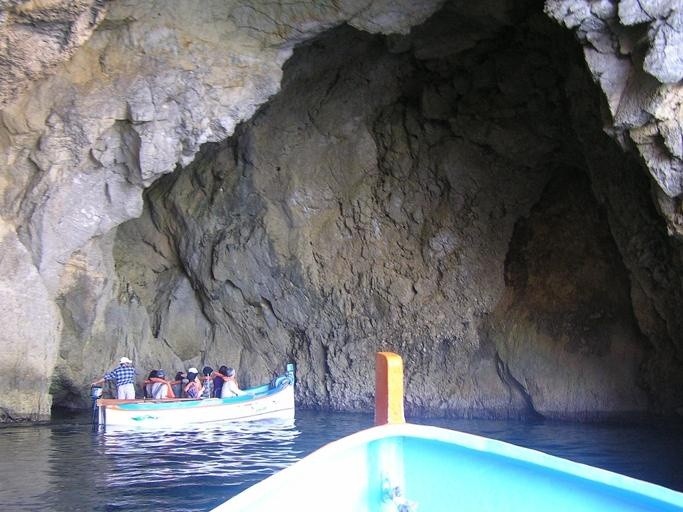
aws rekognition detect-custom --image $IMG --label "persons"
[90,356,136,400]
[143,366,246,399]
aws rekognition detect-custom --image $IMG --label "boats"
[89,362,296,435]
[209,348,682,512]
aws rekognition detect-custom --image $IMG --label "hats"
[156,369,168,377]
[187,372,198,380]
[120,357,132,364]
[203,367,213,376]
[188,368,198,374]
